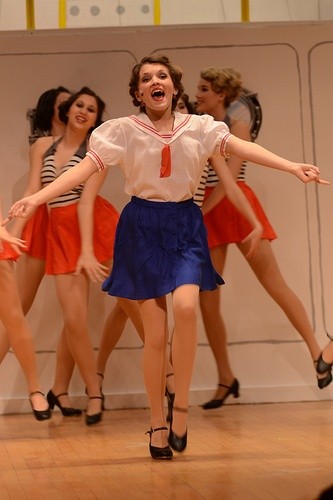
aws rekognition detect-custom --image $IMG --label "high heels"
[313,360,332,390]
[83,394,106,425]
[28,391,53,420]
[47,388,80,415]
[145,428,172,463]
[166,406,189,458]
[201,378,241,413]
[164,371,178,409]
[316,333,332,375]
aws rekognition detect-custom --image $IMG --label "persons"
[0,54,332,500]
[1,55,333,466]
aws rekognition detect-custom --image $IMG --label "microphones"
[139,89,143,96]
